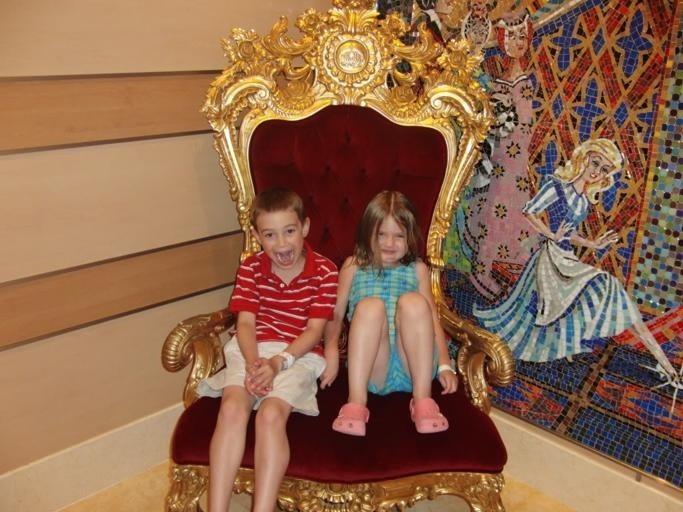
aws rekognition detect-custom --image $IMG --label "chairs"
[161,0,517,512]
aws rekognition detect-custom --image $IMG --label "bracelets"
[436,364,456,376]
[277,350,295,370]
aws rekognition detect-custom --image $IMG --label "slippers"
[410,398,450,433]
[333,403,370,436]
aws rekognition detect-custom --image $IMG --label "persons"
[475,16,547,295]
[318,189,461,438]
[207,188,339,511]
[468,136,681,394]
